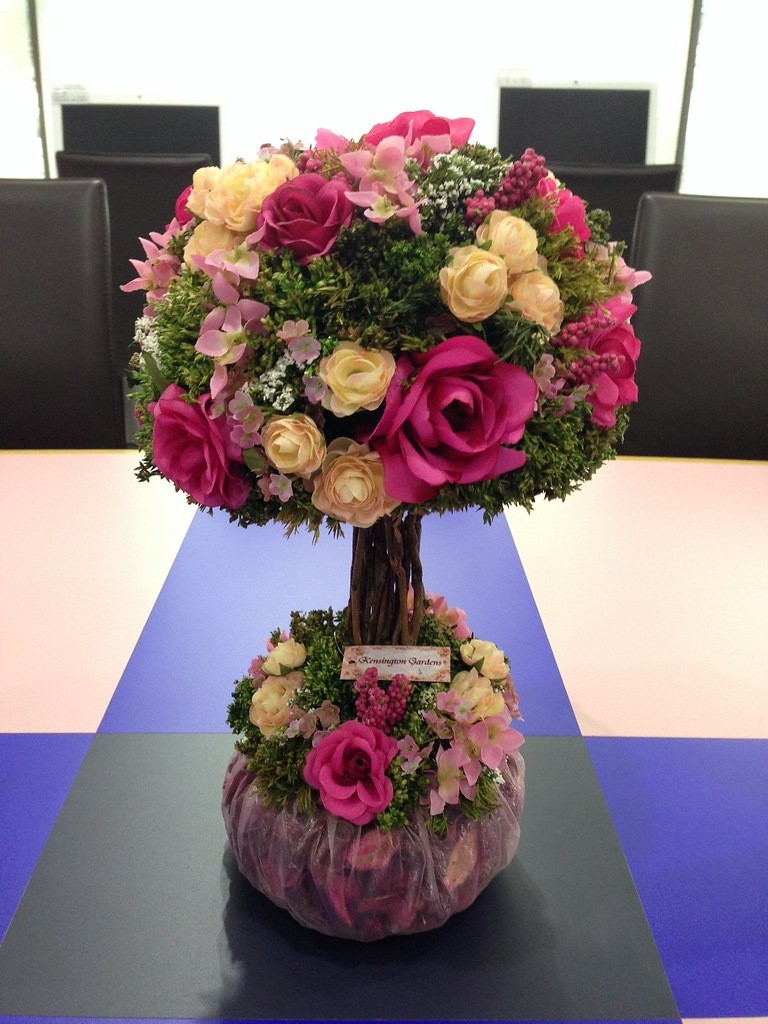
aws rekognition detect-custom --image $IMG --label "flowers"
[117,108,654,645]
[220,591,528,939]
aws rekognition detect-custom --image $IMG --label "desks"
[0,449,768,1024]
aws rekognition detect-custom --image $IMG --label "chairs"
[0,105,223,452]
[495,86,768,462]
